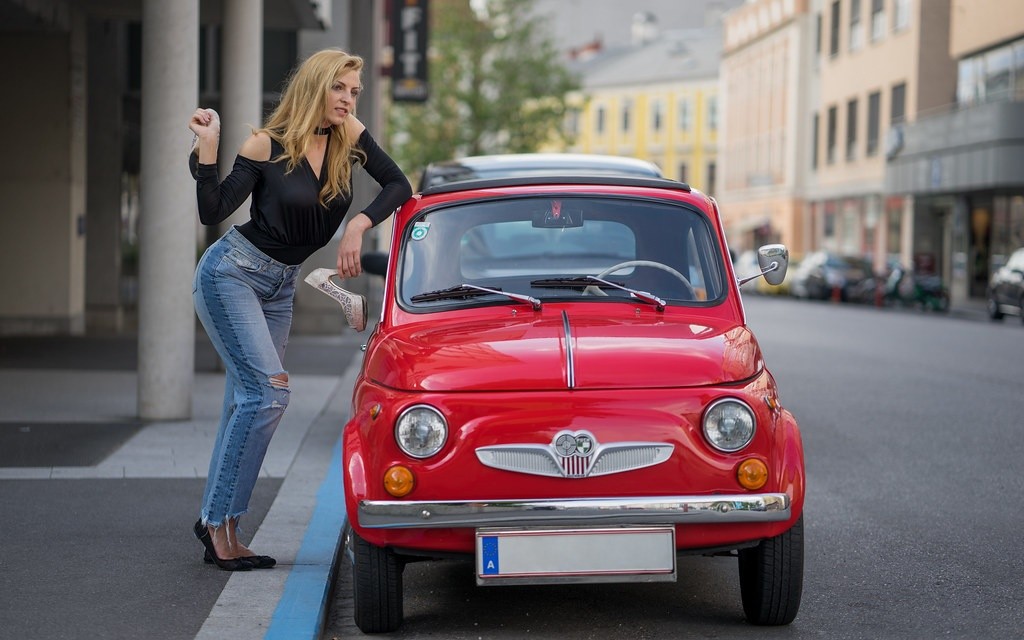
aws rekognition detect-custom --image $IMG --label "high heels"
[305,270,367,332]
[189,107,221,181]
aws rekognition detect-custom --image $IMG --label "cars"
[359,153,708,315]
[985,248,1024,320]
[342,175,808,635]
[733,249,879,305]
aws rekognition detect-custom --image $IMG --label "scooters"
[875,257,956,312]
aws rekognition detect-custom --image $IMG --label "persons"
[189,50,412,572]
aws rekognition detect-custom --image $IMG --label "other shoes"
[193,518,253,571]
[204,540,277,571]
